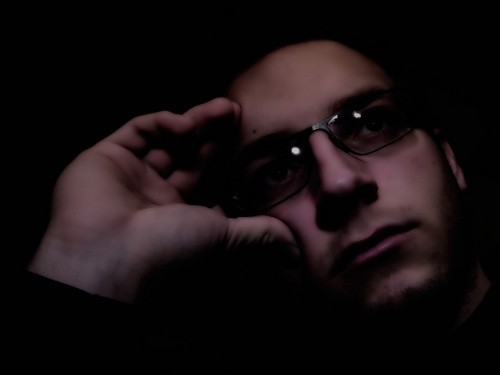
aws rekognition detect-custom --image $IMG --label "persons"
[0,35,500,375]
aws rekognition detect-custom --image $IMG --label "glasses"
[214,95,436,217]
